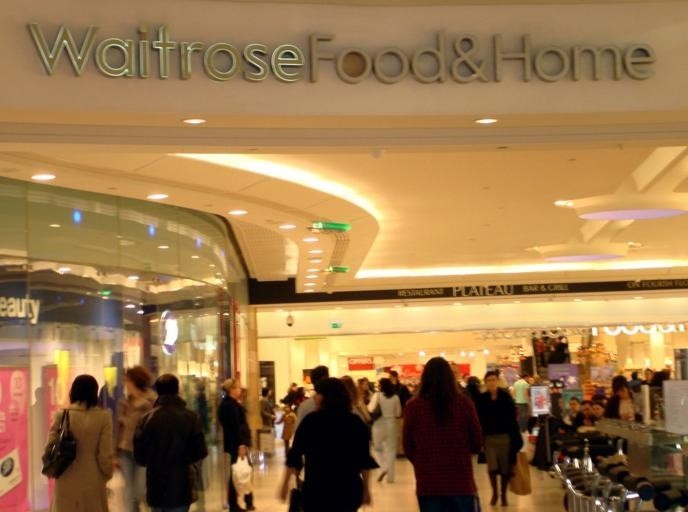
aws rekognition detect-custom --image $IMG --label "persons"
[216,376,259,512]
[257,357,672,511]
[41,372,117,512]
[133,371,209,512]
[30,363,217,481]
[110,364,158,512]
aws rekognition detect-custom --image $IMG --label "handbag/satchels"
[510,452,531,495]
[190,464,199,503]
[42,429,76,480]
[368,403,382,426]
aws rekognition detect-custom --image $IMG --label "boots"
[501,486,507,506]
[491,487,498,505]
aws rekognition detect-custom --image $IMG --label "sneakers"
[247,505,255,510]
[230,505,246,512]
